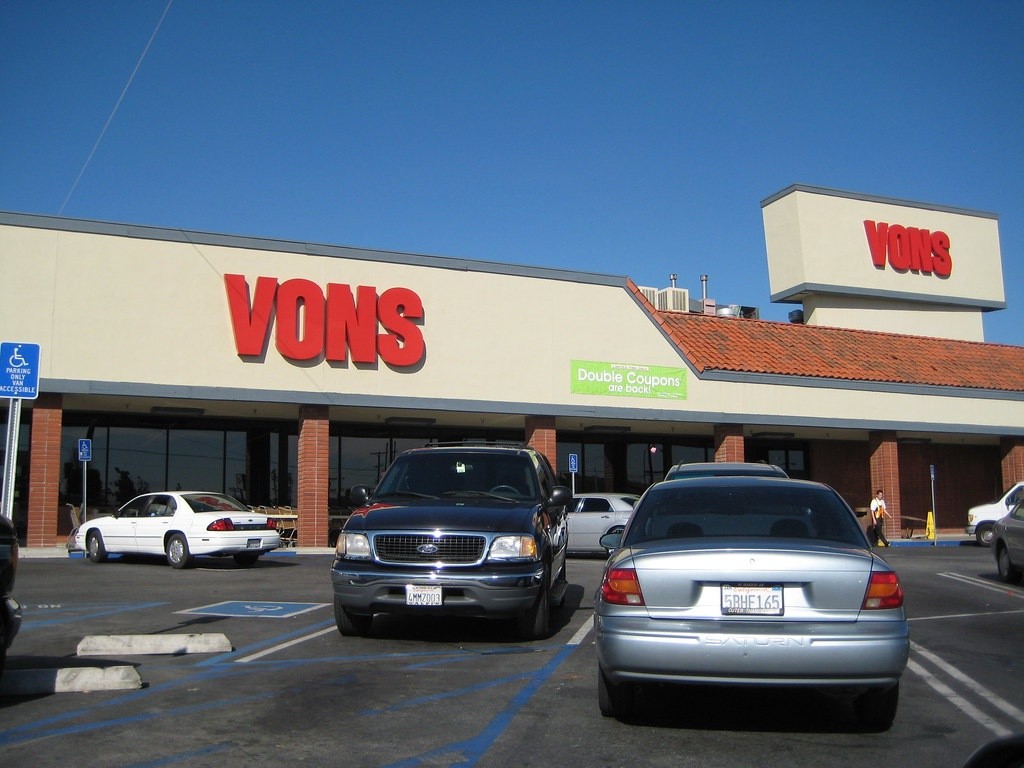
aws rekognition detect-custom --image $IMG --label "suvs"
[644,459,819,542]
[965,481,1024,548]
[331,442,574,640]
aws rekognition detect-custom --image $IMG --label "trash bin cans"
[854,507,873,548]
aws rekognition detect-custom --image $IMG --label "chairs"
[665,523,704,539]
[769,518,810,538]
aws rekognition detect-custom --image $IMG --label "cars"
[559,492,650,557]
[592,477,908,730]
[992,498,1024,583]
[74,491,280,568]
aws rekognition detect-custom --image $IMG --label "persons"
[870,490,892,547]
